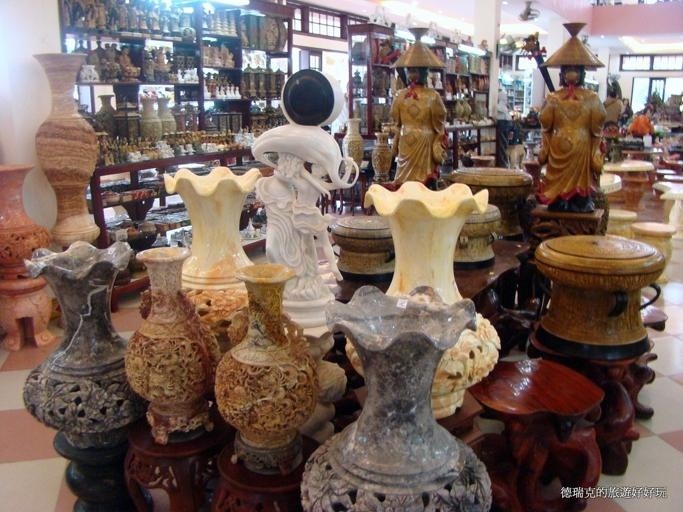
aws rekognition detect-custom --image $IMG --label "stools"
[497,160,682,512]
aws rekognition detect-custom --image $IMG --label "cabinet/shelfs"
[58,0,294,312]
[347,23,533,171]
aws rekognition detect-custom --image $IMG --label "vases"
[35,55,100,245]
[0,119,502,512]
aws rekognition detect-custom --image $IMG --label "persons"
[618,97,632,127]
[537,66,607,214]
[273,157,354,281]
[633,108,654,137]
[385,67,449,193]
[602,90,623,162]
